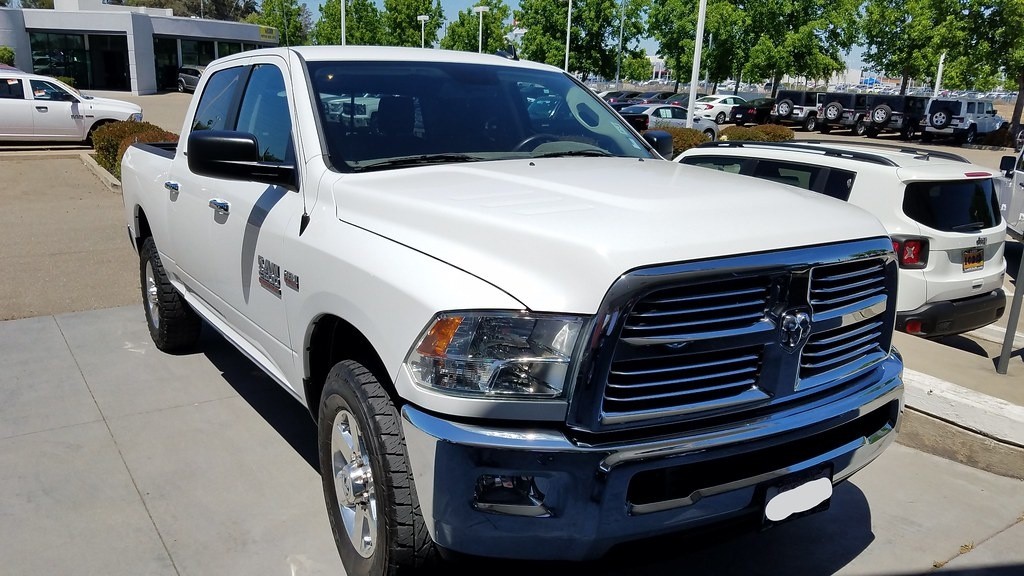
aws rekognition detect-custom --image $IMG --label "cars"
[627,91,677,109]
[0,63,47,97]
[596,90,617,100]
[0,70,144,149]
[729,97,780,127]
[606,91,640,114]
[685,93,748,125]
[659,92,710,110]
[615,103,721,143]
[583,67,1019,107]
[31,47,86,78]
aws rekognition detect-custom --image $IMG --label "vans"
[175,63,207,91]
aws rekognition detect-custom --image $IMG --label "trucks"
[115,42,906,576]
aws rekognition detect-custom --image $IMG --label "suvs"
[992,126,1024,245]
[919,96,1009,145]
[861,93,931,141]
[668,133,1012,341]
[769,90,820,132]
[816,91,872,135]
